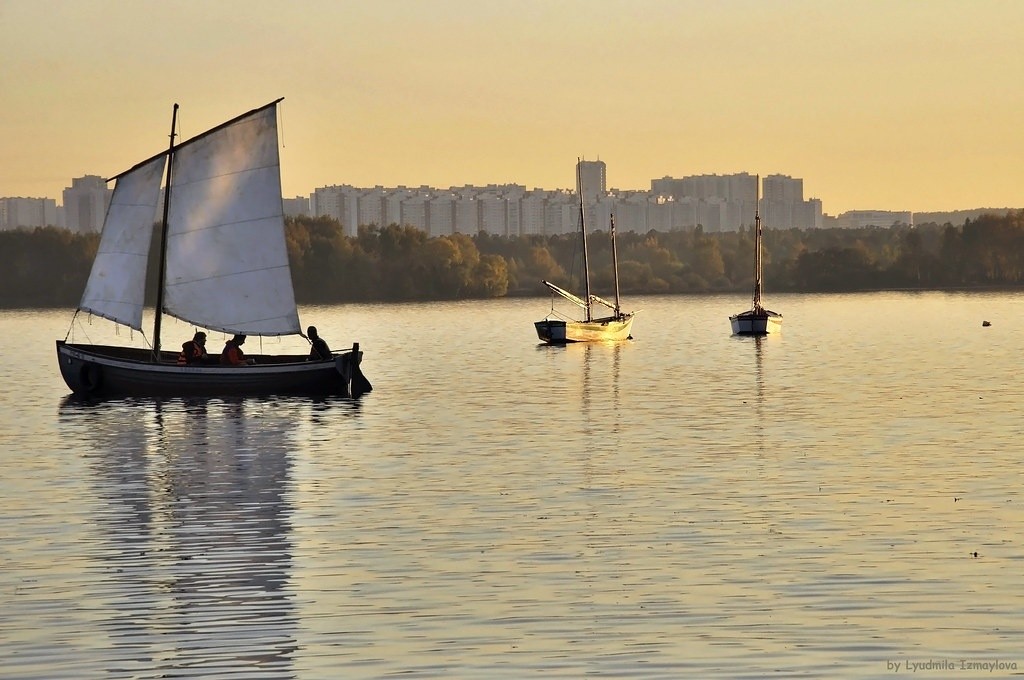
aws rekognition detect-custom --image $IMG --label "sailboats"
[54,90,373,410]
[534,156,645,344]
[729,172,784,336]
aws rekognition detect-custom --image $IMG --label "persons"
[177,332,210,366]
[219,334,250,365]
[306,326,333,361]
